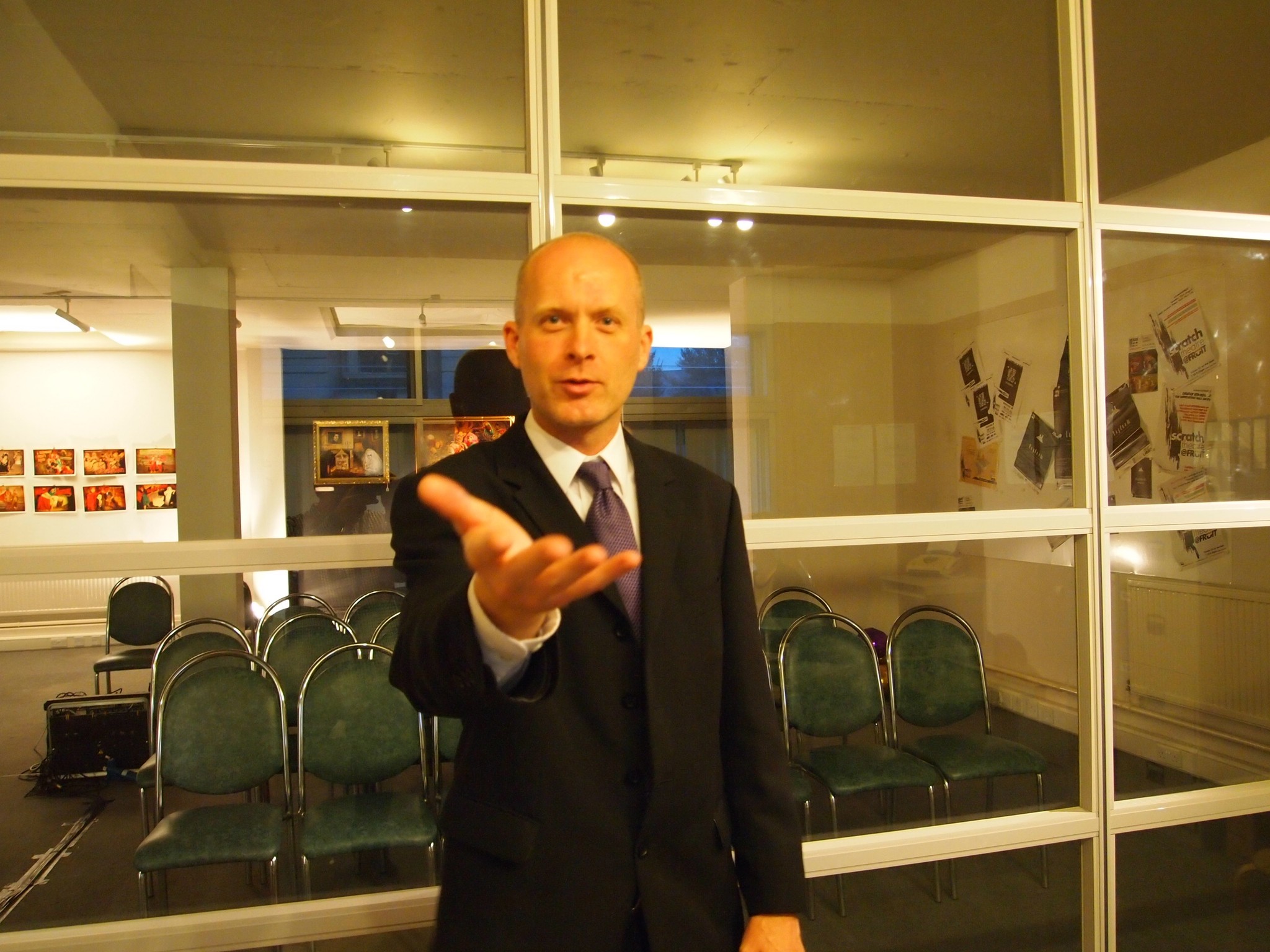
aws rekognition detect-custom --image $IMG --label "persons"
[390,232,804,952]
[449,349,526,443]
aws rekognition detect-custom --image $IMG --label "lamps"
[419,308,426,327]
[55,296,89,333]
[330,144,754,231]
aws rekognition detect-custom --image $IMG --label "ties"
[577,461,641,642]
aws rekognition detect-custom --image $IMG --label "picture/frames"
[416,416,515,475]
[313,420,390,485]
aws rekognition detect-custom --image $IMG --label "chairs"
[92,575,1046,952]
